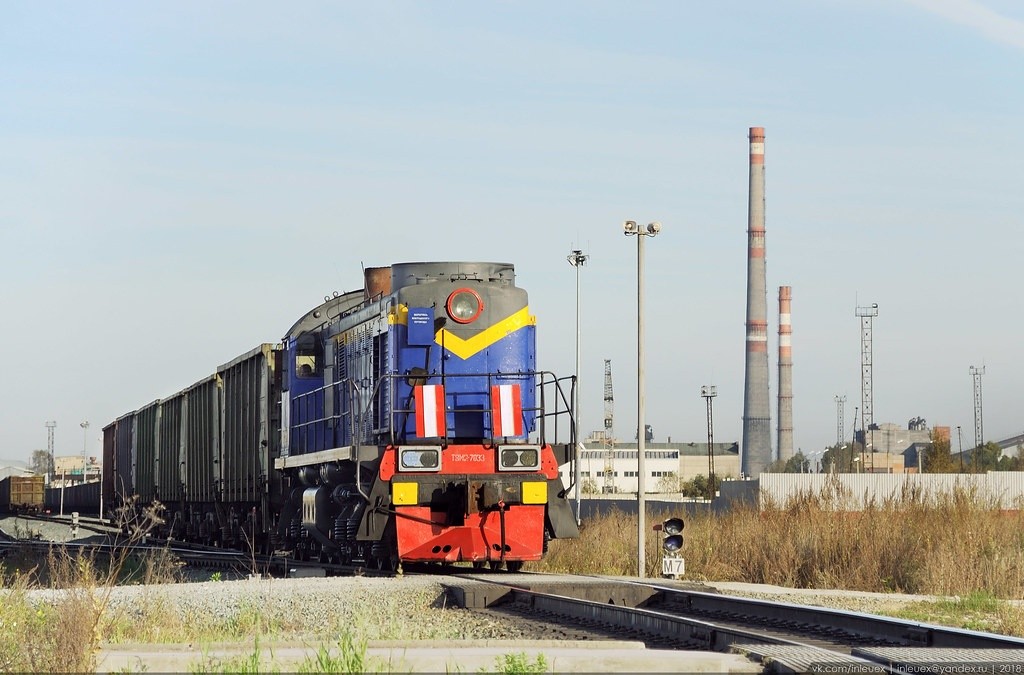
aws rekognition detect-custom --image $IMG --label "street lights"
[957,425,963,470]
[623,220,662,579]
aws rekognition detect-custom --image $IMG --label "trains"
[0,475,47,516]
[96,260,583,569]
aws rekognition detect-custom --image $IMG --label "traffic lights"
[662,517,685,553]
